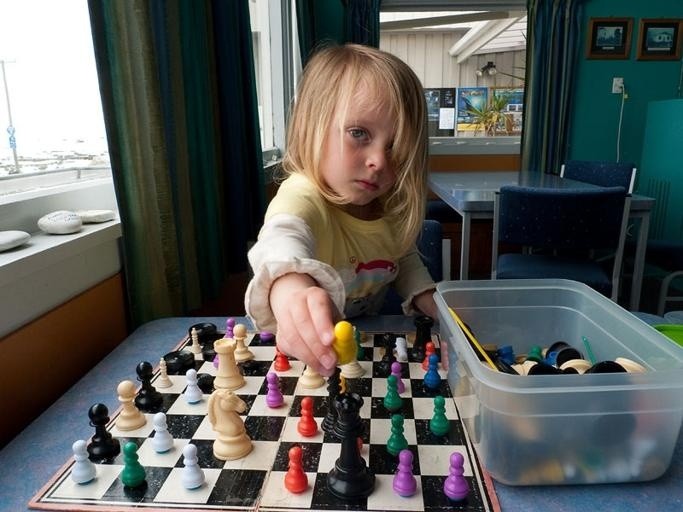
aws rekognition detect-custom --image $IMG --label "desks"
[0,308,682,512]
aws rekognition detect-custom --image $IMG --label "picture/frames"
[585,17,682,62]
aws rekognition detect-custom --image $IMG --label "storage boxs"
[431,274,679,489]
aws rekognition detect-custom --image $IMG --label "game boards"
[29,330,501,507]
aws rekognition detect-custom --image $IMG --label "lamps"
[475,61,497,78]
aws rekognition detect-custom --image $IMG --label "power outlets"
[611,77,624,94]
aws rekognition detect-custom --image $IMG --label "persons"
[241,39,474,380]
